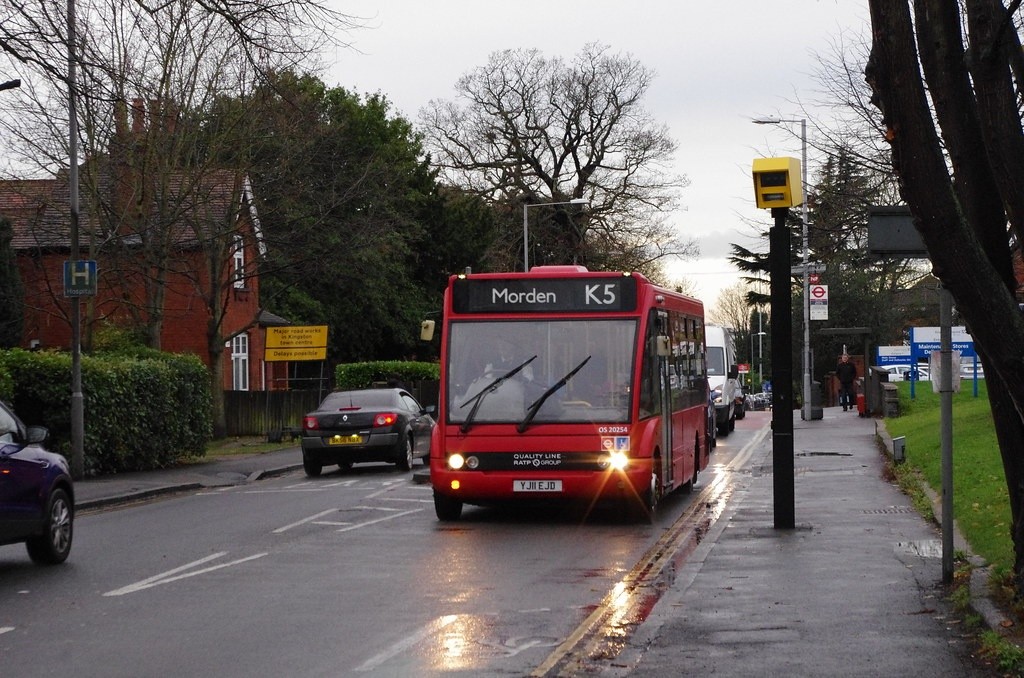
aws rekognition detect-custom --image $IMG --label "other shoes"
[843,405,847,411]
[850,404,853,409]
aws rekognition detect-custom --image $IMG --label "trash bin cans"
[869,365,891,413]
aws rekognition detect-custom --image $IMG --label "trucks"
[704,324,740,438]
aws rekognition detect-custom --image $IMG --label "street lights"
[522,197,593,272]
[753,115,812,422]
[750,332,767,394]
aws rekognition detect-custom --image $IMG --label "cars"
[708,388,723,454]
[0,400,76,567]
[735,379,773,420]
[300,387,437,476]
[869,365,932,382]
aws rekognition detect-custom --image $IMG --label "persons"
[836,354,856,411]
[484,341,534,385]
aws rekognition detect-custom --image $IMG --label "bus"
[419,265,710,525]
